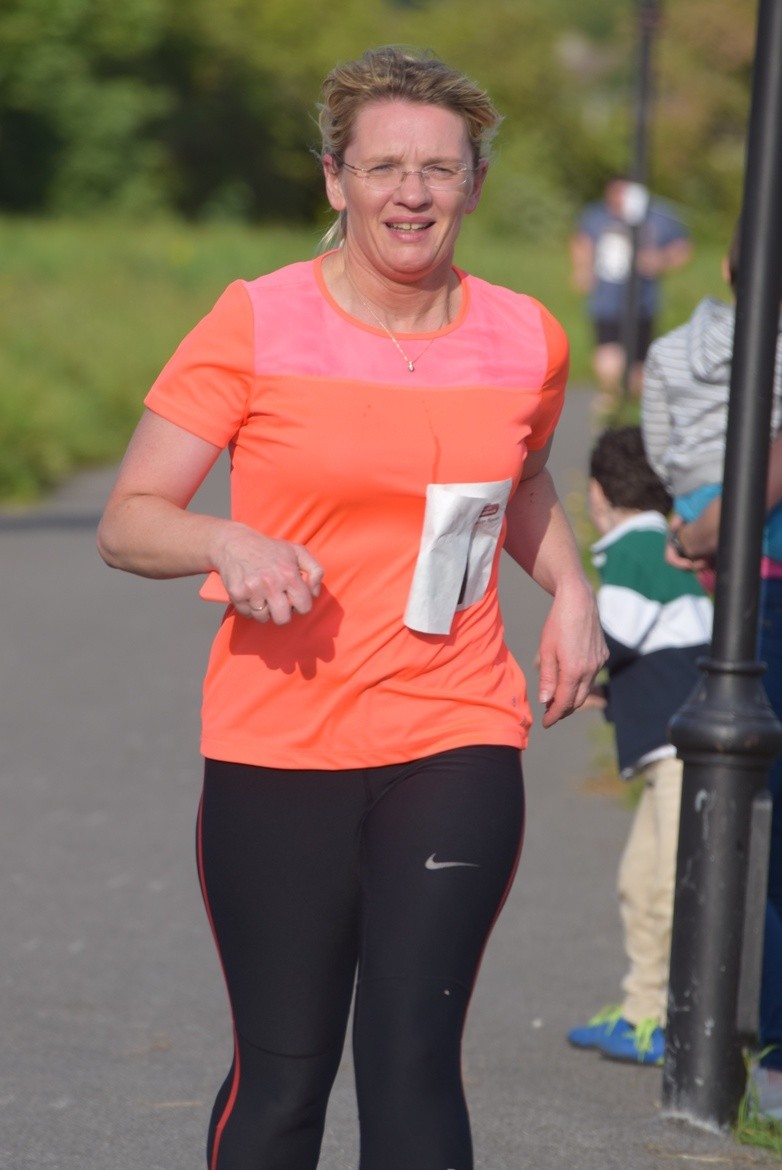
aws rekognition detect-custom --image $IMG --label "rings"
[247,602,267,611]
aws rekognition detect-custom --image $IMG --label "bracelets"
[668,527,706,560]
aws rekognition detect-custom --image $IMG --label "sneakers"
[565,1005,634,1047]
[598,1016,667,1066]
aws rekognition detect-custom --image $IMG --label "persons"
[572,166,690,428]
[566,422,713,1064]
[99,49,612,1170]
[641,224,782,1145]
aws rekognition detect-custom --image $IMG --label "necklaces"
[344,267,449,372]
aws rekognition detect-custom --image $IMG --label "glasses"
[331,152,480,190]
